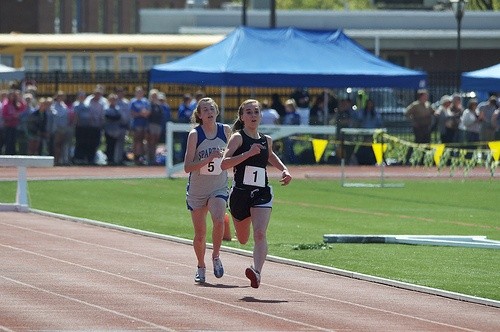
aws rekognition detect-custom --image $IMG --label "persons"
[0,81,384,168]
[183,97,233,284]
[220,99,292,289]
[401,89,500,166]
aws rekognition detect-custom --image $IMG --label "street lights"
[450,0,467,93]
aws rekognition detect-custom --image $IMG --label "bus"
[0,32,340,124]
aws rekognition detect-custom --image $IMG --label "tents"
[148,25,428,140]
[461,63,500,93]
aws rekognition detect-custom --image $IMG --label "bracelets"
[242,153,247,158]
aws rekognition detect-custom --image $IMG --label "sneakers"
[212,254,224,278]
[194,264,206,283]
[245,265,261,288]
